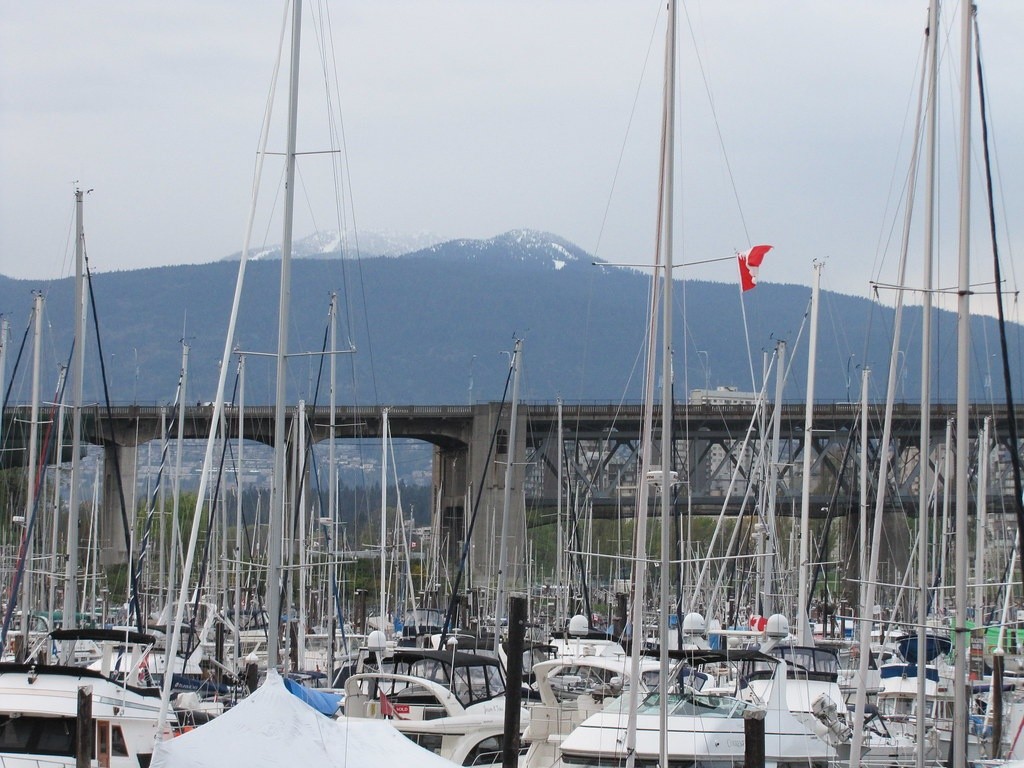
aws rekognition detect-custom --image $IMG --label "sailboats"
[0,0,1024,768]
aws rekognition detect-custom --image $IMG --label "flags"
[737,245,773,291]
